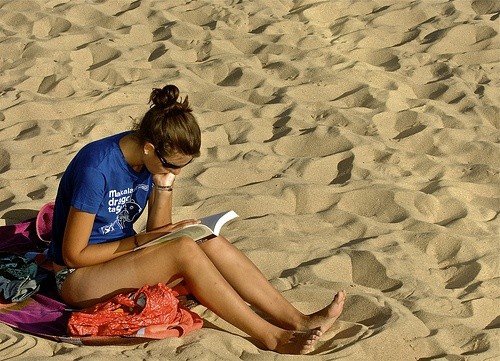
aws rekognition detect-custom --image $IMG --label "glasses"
[154,147,193,168]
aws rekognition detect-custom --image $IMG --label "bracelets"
[134,234,140,248]
[151,183,174,191]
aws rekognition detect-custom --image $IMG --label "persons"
[49,85,346,354]
